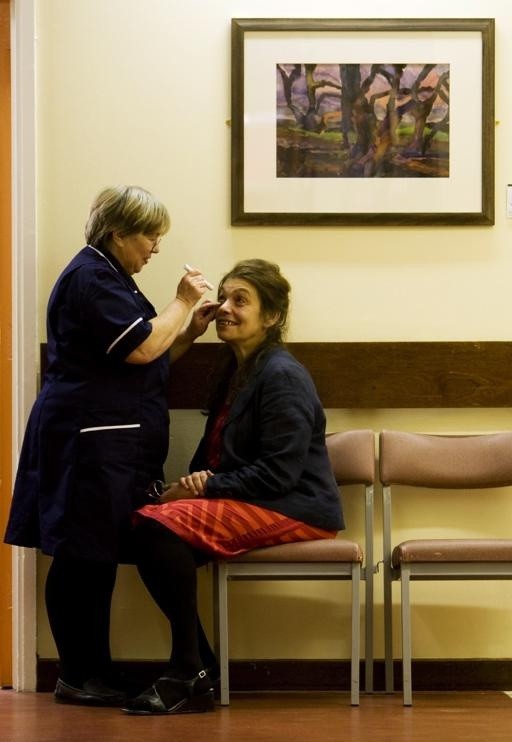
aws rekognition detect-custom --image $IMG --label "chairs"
[219,427,375,706]
[380,429,510,708]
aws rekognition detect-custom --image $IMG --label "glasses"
[145,479,166,501]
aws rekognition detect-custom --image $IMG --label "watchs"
[4,184,221,706]
[119,259,347,715]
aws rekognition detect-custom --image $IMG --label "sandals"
[49,660,221,715]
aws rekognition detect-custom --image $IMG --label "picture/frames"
[229,14,500,229]
